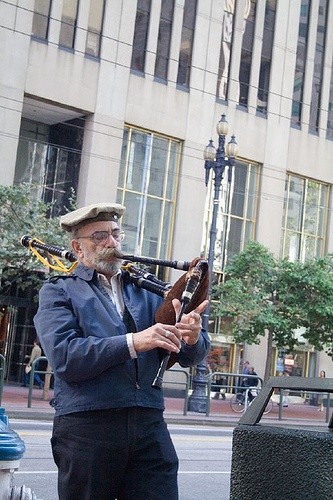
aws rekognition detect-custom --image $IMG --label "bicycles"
[230,392,272,415]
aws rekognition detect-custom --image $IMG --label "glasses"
[76,230,126,242]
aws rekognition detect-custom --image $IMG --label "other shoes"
[277,403,280,405]
[283,404,288,407]
[20,384,29,387]
[39,381,44,389]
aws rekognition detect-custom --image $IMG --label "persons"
[21,341,45,390]
[32,203,211,500]
[316,371,326,413]
[235,360,258,405]
[212,364,227,400]
[277,370,291,408]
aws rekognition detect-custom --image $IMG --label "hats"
[59,203,126,232]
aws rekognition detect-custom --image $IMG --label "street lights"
[187,111,237,413]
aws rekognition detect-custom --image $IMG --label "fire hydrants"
[0,407,39,500]
